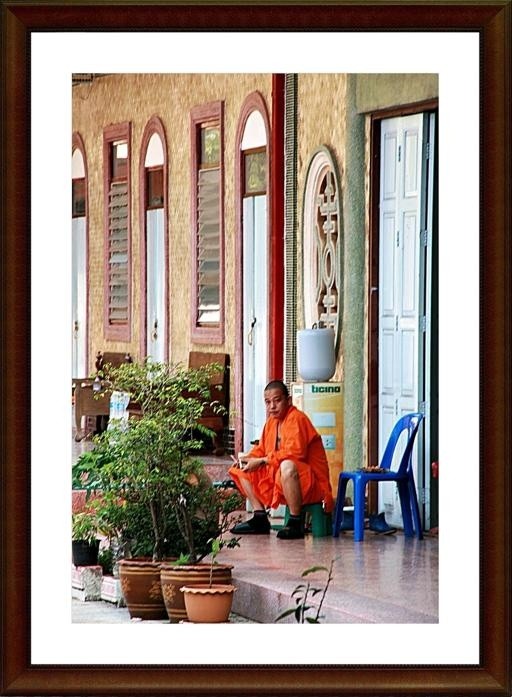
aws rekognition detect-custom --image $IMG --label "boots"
[368,512,397,536]
[339,511,354,534]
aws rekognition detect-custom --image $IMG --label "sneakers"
[230,521,270,535]
[276,527,305,540]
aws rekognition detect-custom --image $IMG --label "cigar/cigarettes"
[229,454,238,463]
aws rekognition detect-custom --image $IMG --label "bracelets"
[261,459,265,464]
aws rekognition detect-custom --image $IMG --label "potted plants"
[72,356,238,621]
[72,511,101,567]
[179,539,237,623]
[145,470,247,624]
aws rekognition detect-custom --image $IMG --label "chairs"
[182,352,230,456]
[72,351,133,442]
[332,412,424,542]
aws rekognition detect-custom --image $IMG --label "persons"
[228,380,335,539]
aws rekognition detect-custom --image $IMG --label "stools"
[284,502,331,537]
